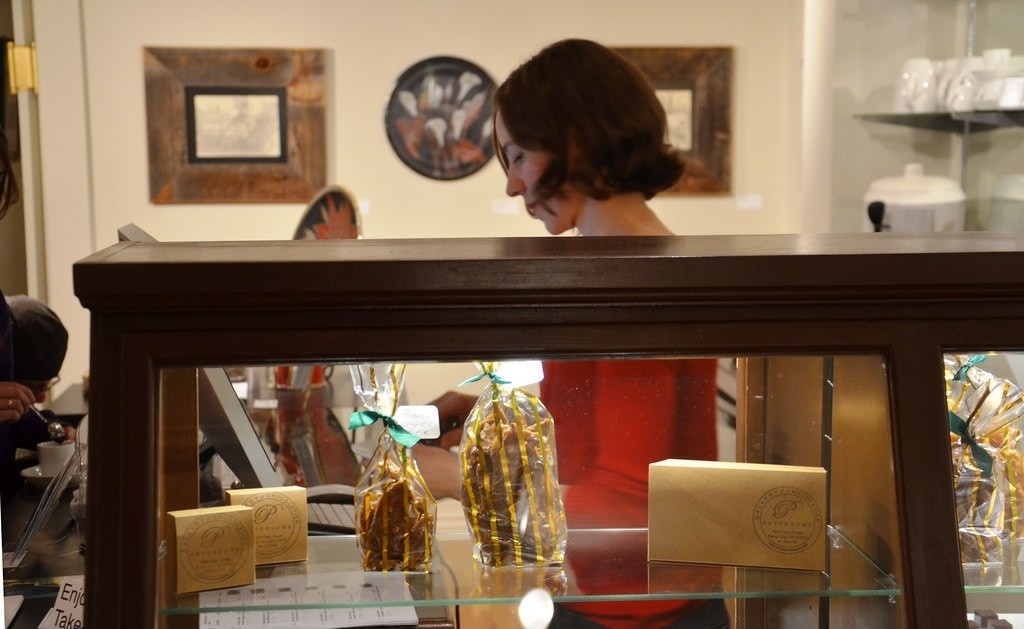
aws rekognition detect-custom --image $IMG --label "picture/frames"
[144,44,329,206]
[607,42,734,198]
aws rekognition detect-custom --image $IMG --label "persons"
[0,132,77,448]
[362,37,733,629]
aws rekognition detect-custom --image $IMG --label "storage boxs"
[167,507,257,597]
[647,459,827,570]
[225,485,307,564]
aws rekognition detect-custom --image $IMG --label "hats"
[0,295,69,381]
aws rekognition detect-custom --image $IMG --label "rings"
[8,398,13,409]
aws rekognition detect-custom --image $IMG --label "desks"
[1,379,454,628]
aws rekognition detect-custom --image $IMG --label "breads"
[359,412,563,571]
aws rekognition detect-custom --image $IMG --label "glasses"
[25,377,58,388]
